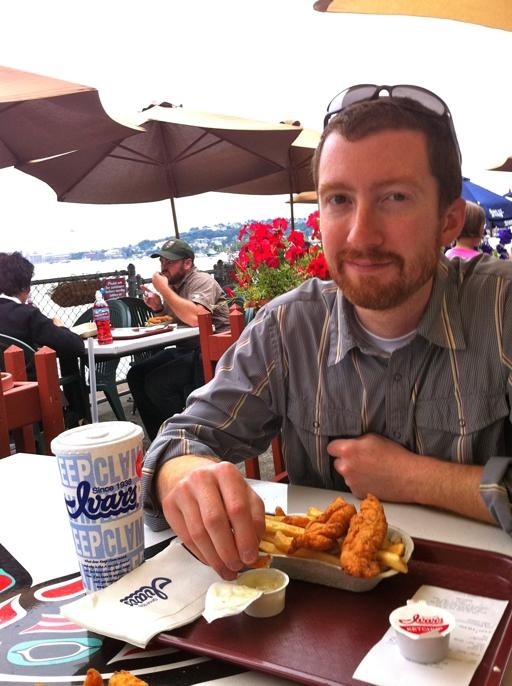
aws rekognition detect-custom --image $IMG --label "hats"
[149,239,194,261]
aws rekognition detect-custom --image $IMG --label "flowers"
[224,205,331,309]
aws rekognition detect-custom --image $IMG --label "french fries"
[258,507,409,575]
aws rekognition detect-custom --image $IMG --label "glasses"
[324,84,461,164]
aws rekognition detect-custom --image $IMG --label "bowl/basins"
[147,320,173,327]
[253,513,415,592]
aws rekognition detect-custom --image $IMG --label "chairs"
[0,297,157,421]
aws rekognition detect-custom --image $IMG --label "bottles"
[92,290,113,345]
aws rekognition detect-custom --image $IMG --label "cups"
[50,421,144,595]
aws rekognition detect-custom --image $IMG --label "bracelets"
[154,305,164,314]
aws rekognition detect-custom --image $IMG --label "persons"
[124,238,232,443]
[443,201,489,263]
[136,93,512,585]
[0,250,88,454]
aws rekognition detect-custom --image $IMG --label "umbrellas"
[311,1,512,33]
[0,63,134,172]
[11,101,304,241]
[208,124,328,236]
[484,156,512,175]
[458,174,512,221]
[284,191,320,208]
